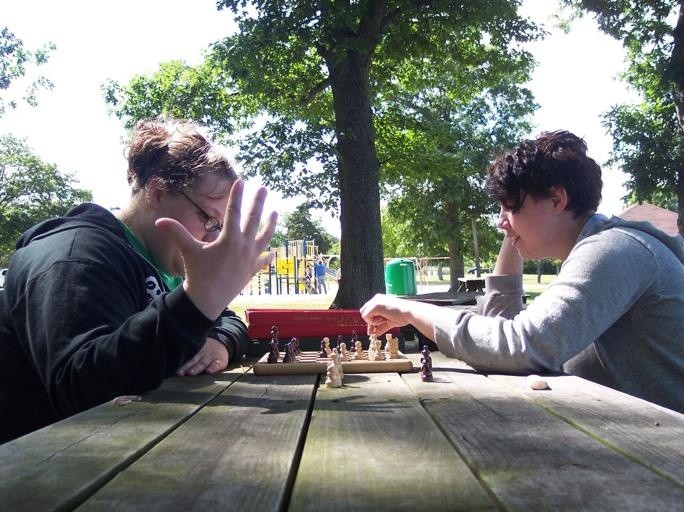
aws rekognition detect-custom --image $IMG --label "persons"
[0,117,279,446]
[358,129,682,415]
[303,258,328,295]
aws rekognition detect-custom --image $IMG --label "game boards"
[253,347,413,373]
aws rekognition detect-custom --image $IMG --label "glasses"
[179,188,223,232]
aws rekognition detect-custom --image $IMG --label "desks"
[0,351,684,512]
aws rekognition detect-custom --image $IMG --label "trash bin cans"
[384,259,417,296]
